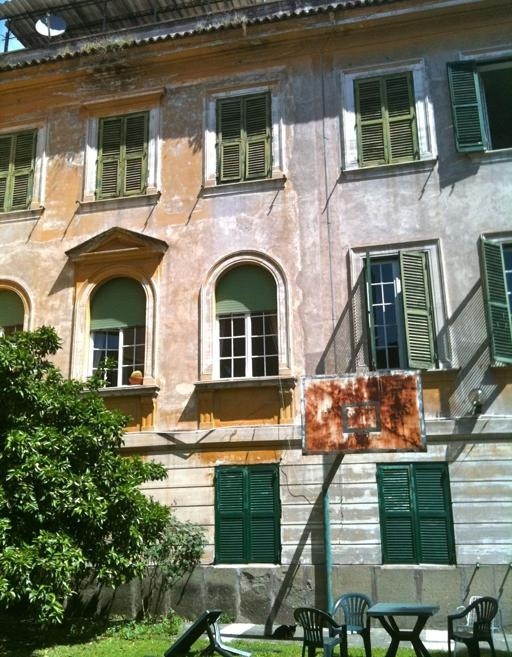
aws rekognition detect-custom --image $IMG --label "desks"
[366,603,441,656]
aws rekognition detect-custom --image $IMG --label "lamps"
[469,389,487,414]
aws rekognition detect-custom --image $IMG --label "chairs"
[447,595,499,657]
[294,593,372,656]
